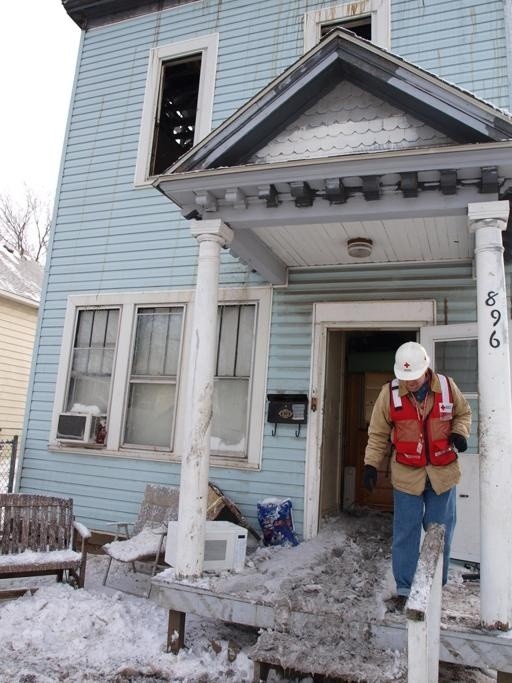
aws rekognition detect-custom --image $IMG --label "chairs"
[99,484,184,598]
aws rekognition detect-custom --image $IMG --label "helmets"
[394,341,430,380]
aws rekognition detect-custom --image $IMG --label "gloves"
[364,464,378,490]
[448,432,468,452]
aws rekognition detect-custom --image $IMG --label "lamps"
[347,237,373,258]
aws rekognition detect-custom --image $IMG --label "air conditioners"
[54,412,107,444]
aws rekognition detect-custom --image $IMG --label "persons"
[361,342,472,613]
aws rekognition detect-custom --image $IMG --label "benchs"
[0,491,92,596]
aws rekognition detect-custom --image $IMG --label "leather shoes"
[396,595,407,610]
